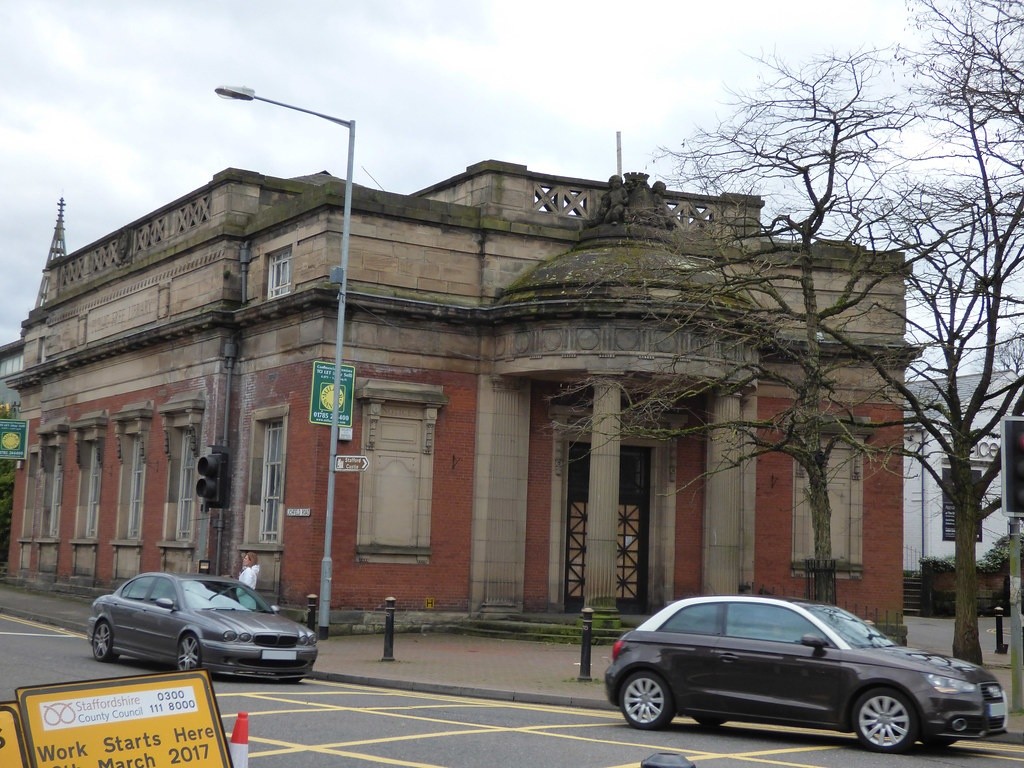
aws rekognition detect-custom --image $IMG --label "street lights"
[213,85,359,641]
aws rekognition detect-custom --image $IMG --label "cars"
[86,572,322,685]
[603,594,1009,756]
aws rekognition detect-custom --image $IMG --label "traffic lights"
[193,451,228,507]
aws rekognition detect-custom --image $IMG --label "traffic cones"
[227,712,253,768]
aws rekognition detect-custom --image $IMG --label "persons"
[236,552,260,612]
